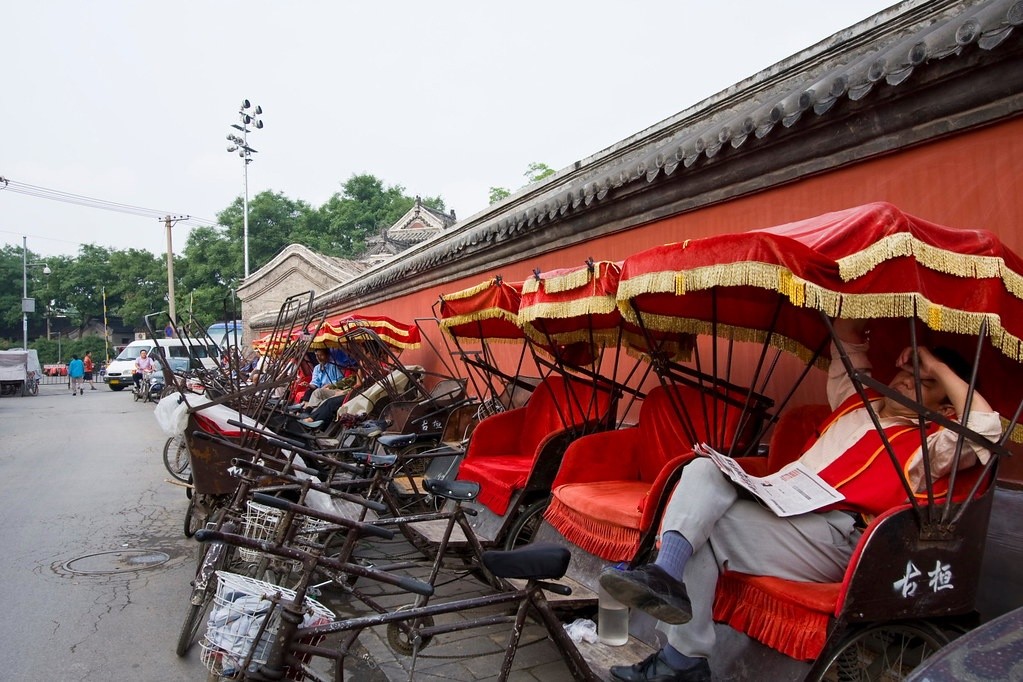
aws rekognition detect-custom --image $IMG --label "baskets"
[238,500,318,574]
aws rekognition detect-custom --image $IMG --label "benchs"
[541,383,769,562]
[454,376,619,514]
[668,403,991,662]
[335,364,544,455]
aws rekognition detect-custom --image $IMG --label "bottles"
[293,404,301,417]
[598,562,628,646]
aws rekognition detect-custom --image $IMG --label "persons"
[301,340,390,438]
[598,317,1002,682]
[221,345,319,405]
[68,354,84,396]
[189,354,201,369]
[133,350,154,402]
[299,348,344,412]
[77,350,97,390]
[294,351,389,418]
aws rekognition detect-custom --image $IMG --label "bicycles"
[133,367,152,403]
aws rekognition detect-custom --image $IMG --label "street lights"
[225,97,264,278]
[21,235,53,350]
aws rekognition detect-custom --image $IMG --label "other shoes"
[73,392,77,395]
[91,387,97,390]
[80,388,83,395]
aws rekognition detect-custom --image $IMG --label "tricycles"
[142,281,1023,682]
[0,346,43,398]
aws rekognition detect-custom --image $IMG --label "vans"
[206,317,243,365]
[102,336,221,393]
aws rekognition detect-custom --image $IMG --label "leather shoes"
[608,649,712,682]
[599,562,693,625]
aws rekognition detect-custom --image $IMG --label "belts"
[197,569,337,682]
